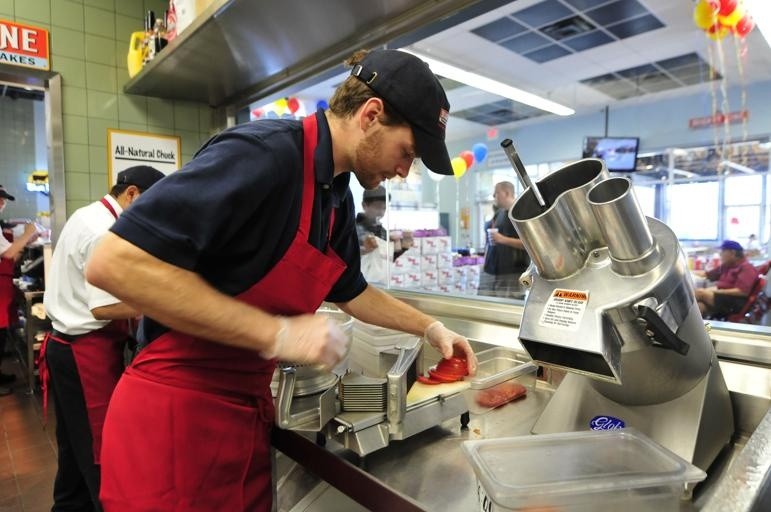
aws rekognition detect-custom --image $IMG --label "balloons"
[428,142,488,183]
[693,0,755,41]
[315,99,327,115]
[247,96,300,121]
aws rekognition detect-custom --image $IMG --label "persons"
[35,165,168,512]
[354,182,414,288]
[0,183,40,397]
[479,180,532,300]
[84,45,479,512]
[690,240,759,320]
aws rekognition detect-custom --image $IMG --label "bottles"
[140,9,169,69]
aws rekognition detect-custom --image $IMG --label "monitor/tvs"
[582,137,639,172]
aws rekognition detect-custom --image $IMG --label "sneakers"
[1,385,13,396]
[1,373,17,384]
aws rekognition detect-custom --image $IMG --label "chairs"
[709,275,765,322]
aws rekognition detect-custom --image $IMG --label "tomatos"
[417,355,468,385]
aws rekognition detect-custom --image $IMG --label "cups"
[487,228,499,246]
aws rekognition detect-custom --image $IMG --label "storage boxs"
[460,427,707,512]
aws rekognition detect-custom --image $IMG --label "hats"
[0,185,15,201]
[0,219,17,229]
[351,50,454,175]
[715,240,741,250]
[118,166,164,190]
